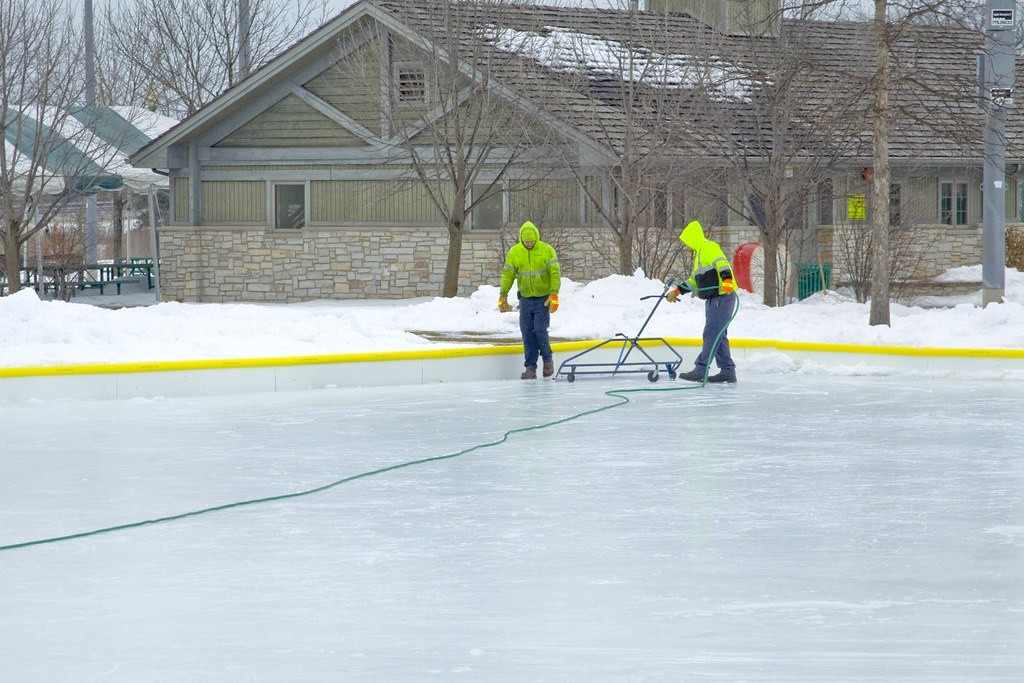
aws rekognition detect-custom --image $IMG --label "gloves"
[544,295,558,313]
[720,279,732,293]
[666,288,681,303]
[498,296,507,313]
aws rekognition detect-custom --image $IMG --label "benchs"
[0,269,161,295]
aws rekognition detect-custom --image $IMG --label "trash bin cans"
[794,261,834,301]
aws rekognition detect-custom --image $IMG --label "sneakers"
[679,368,706,383]
[542,359,554,376]
[707,374,737,383]
[521,367,537,379]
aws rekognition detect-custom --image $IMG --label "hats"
[521,228,536,242]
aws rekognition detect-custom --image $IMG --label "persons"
[498,221,561,379]
[666,220,739,383]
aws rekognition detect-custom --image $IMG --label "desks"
[22,257,160,295]
